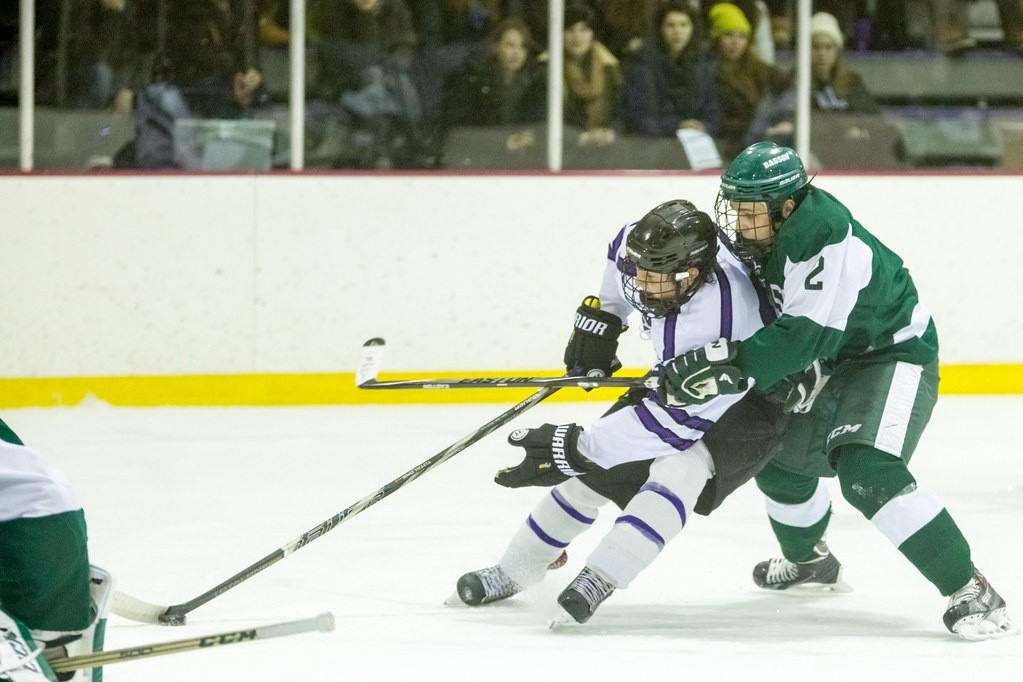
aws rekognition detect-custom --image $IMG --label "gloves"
[562,295,622,390]
[643,337,749,409]
[493,423,583,488]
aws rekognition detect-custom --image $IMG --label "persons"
[438,199,793,631]
[32,0,1023,177]
[649,141,1007,641]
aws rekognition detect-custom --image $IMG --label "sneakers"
[440,549,568,607]
[548,564,616,629]
[943,561,1019,639]
[752,540,840,591]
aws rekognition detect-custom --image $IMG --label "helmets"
[712,141,808,273]
[623,199,716,321]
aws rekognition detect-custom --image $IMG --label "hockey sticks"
[354,336,755,392]
[88,353,622,627]
[47,610,336,674]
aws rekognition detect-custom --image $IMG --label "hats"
[707,2,751,39]
[811,12,843,47]
